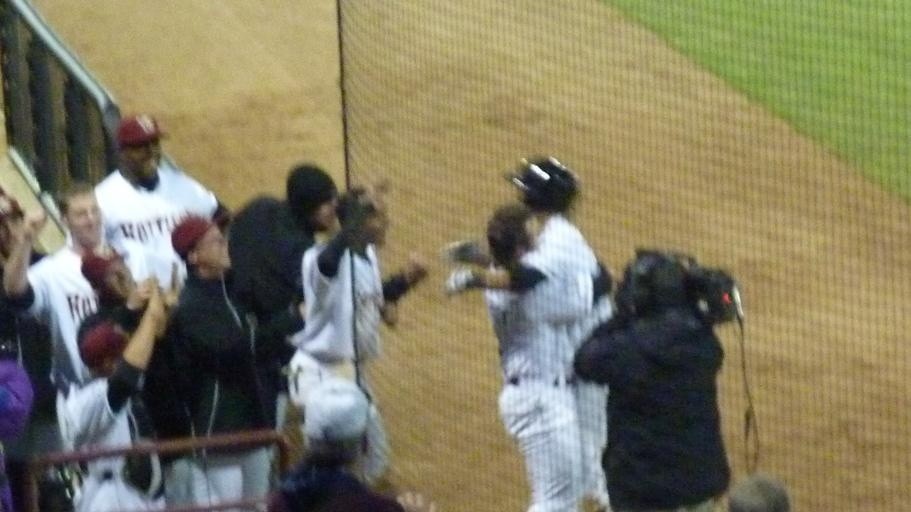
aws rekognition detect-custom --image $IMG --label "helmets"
[500,153,583,212]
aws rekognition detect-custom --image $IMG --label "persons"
[480,200,616,512]
[439,152,617,512]
[0,112,428,512]
[570,246,738,511]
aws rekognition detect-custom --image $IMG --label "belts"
[510,376,577,388]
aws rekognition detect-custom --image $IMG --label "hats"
[78,241,129,290]
[78,315,137,367]
[117,112,168,150]
[171,210,232,258]
